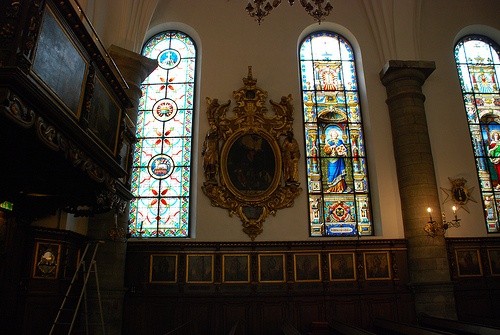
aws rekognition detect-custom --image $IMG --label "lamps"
[244,0,334,27]
[423,204,462,239]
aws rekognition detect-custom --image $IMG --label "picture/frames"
[328,252,357,281]
[30,242,61,280]
[454,249,484,277]
[487,248,500,276]
[363,251,392,281]
[221,254,251,284]
[185,254,214,284]
[149,254,178,284]
[293,253,322,283]
[257,253,286,283]
[200,65,304,241]
